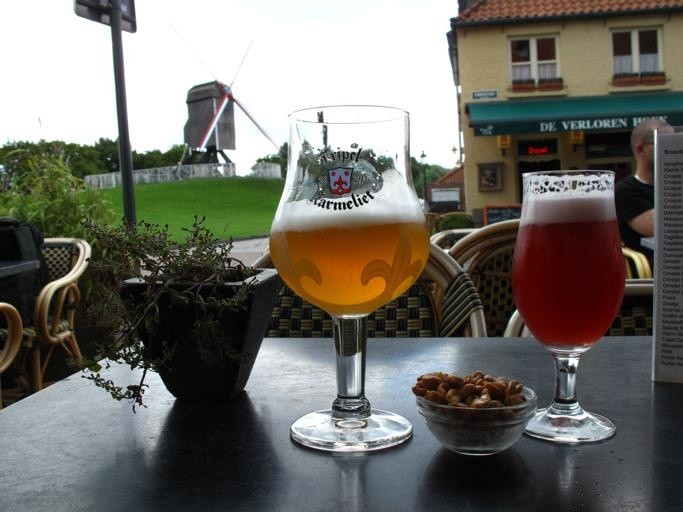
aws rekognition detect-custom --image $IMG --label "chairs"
[0,300,25,374]
[425,226,476,252]
[498,274,655,338]
[443,216,647,337]
[618,236,653,280]
[247,233,495,340]
[0,232,94,408]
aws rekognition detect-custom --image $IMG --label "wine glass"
[512,167,627,443]
[268,101,429,456]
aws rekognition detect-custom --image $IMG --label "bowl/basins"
[415,378,539,456]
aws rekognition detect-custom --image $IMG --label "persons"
[614,119,674,279]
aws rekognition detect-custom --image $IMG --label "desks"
[638,233,657,251]
[0,332,683,511]
[0,258,39,281]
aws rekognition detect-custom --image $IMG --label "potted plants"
[54,192,285,415]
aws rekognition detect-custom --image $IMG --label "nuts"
[412,373,527,420]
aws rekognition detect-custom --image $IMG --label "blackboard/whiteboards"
[484,203,521,226]
